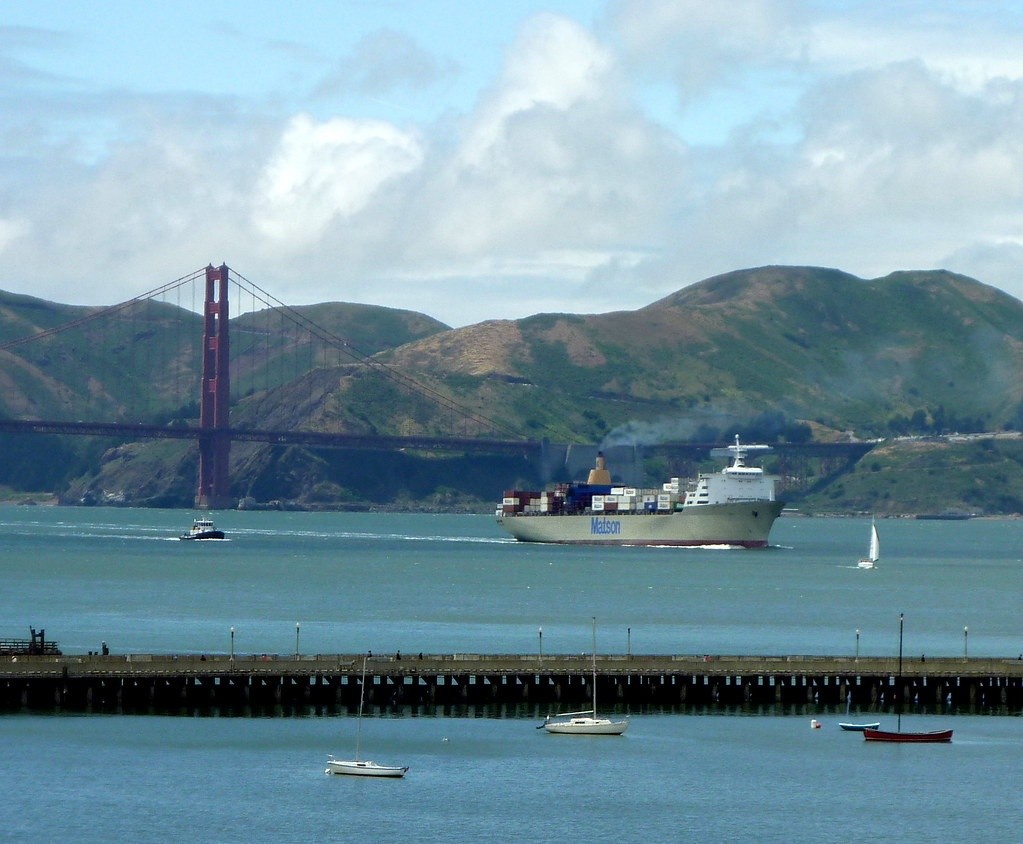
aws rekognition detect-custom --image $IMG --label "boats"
[177,514,225,543]
[493,432,787,552]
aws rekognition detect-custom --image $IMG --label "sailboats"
[323,655,410,778]
[535,615,630,738]
[839,629,880,733]
[858,515,881,569]
[864,614,956,743]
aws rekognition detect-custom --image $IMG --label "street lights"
[294,622,300,663]
[627,627,632,663]
[538,626,542,664]
[854,627,861,663]
[230,626,236,674]
[963,625,969,665]
